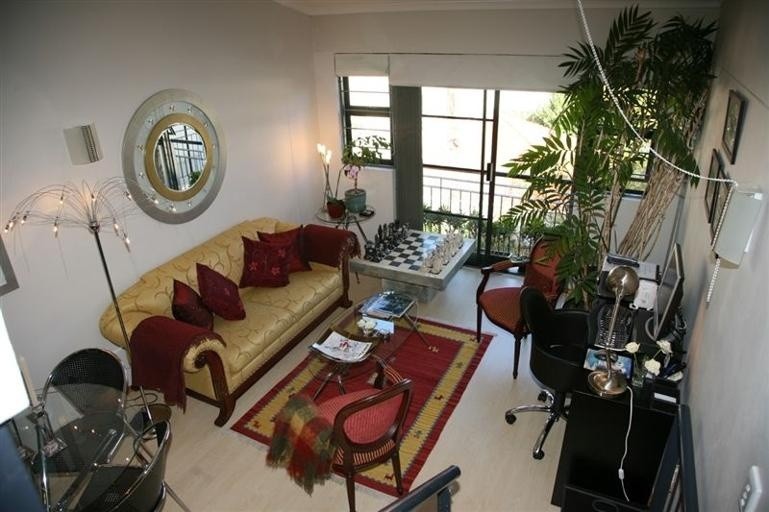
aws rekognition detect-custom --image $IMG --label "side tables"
[316,205,376,242]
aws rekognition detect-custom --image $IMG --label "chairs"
[475,234,575,379]
[505,286,591,460]
[75,420,191,512]
[379,464,462,512]
[317,366,414,512]
[30,348,129,476]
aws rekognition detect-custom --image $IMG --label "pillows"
[172,278,214,330]
[196,262,246,321]
[239,235,293,289]
[257,224,313,273]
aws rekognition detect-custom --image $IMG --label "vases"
[345,188,366,212]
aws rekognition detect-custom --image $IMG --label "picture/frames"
[710,170,730,245]
[721,90,746,164]
[703,148,721,224]
[0,234,20,297]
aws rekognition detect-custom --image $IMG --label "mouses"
[594,348,618,362]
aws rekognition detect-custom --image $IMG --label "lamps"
[317,143,334,211]
[714,183,763,266]
[63,121,103,166]
[587,264,639,398]
[4,178,176,433]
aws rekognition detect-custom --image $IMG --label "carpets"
[229,305,494,499]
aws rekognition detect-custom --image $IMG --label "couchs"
[99,217,355,427]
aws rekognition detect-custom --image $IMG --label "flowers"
[344,160,361,194]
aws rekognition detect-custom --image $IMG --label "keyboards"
[595,303,632,352]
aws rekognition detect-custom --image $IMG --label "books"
[358,290,415,320]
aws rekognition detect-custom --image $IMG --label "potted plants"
[327,136,392,218]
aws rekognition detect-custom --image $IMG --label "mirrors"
[122,89,227,225]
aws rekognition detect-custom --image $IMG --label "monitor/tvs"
[644,243,683,343]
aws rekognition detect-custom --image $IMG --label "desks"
[551,298,680,508]
[349,229,477,304]
